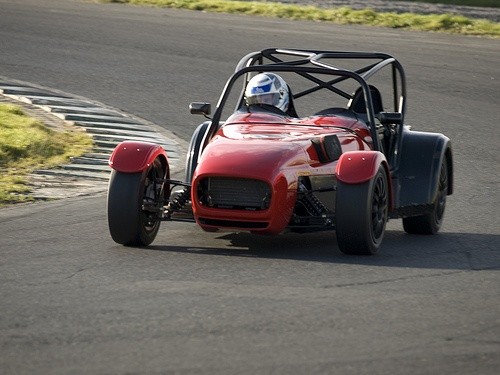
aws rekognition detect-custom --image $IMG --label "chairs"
[348,85,383,113]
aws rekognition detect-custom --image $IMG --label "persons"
[242,72,290,113]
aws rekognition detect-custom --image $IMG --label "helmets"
[244,72,290,113]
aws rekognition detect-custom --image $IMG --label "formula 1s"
[107,46,453,254]
